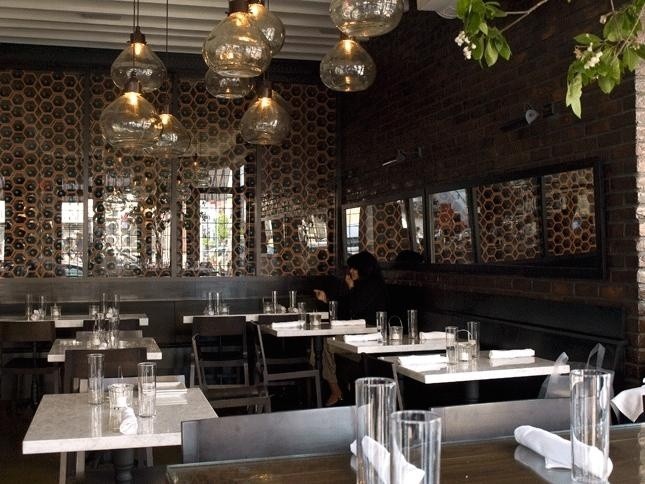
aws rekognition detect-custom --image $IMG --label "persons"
[312,250,401,407]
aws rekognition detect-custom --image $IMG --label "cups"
[84,291,157,420]
[24,291,62,322]
[568,368,615,484]
[262,289,339,327]
[202,290,231,316]
[354,377,444,483]
[445,321,480,364]
[375,307,421,344]
